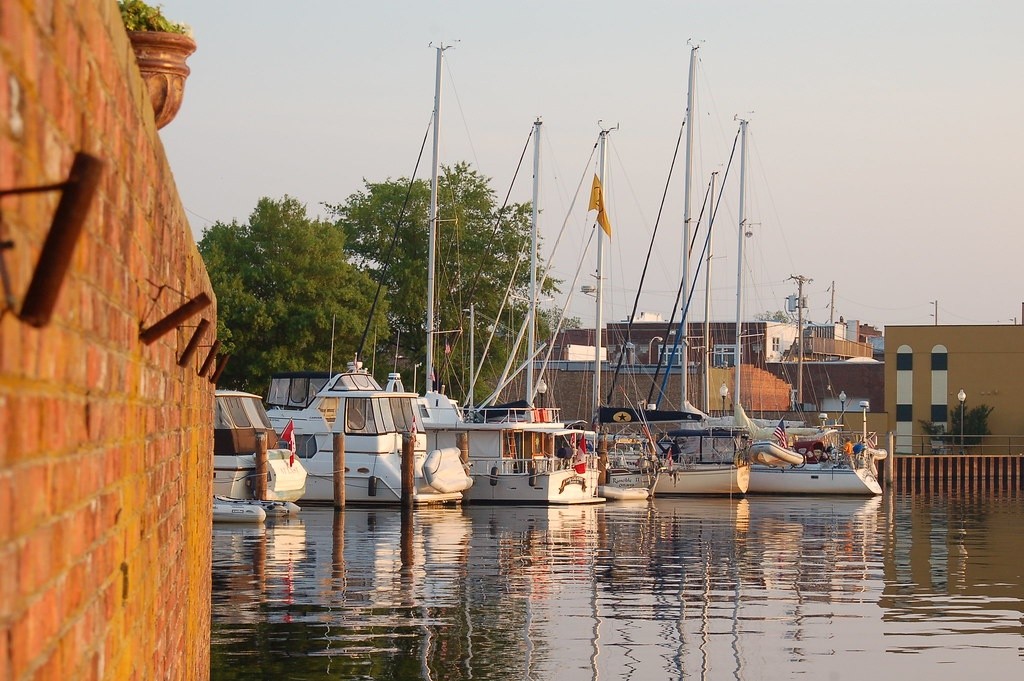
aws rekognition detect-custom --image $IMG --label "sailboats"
[263,36,884,504]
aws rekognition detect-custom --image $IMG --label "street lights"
[957,389,966,455]
[537,379,547,408]
[838,391,846,443]
[648,335,663,365]
[719,380,728,417]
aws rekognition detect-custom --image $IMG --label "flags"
[771,419,788,450]
[587,174,611,240]
[666,448,673,472]
[278,420,296,467]
[573,434,586,474]
[570,433,575,449]
[430,363,435,381]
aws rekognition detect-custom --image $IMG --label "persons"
[841,437,854,470]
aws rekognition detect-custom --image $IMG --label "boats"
[212,494,302,521]
[213,389,308,502]
